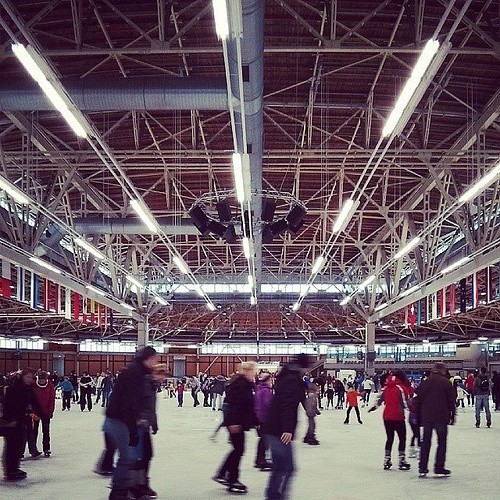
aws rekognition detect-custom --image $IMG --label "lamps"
[212,0,230,41]
[11,43,94,138]
[381,39,439,138]
[229,153,244,203]
[0,174,36,205]
[458,164,500,203]
[29,198,470,312]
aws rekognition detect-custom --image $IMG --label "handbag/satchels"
[0,416,20,436]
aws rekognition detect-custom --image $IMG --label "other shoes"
[95,464,116,476]
[255,462,273,471]
[44,450,51,456]
[304,434,319,444]
[68,408,70,411]
[19,455,24,461]
[62,408,65,411]
[32,452,42,460]
[409,446,421,459]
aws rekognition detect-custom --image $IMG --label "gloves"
[151,422,158,435]
[368,406,377,412]
[128,431,139,447]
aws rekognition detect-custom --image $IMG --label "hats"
[215,375,226,381]
[258,372,270,380]
[431,362,446,371]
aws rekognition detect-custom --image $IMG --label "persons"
[0,347,500,500]
[261,353,311,500]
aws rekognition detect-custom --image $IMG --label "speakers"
[189,197,306,244]
[357,351,363,360]
[368,351,376,360]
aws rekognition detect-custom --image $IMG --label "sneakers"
[487,421,491,427]
[109,483,157,500]
[383,456,392,469]
[228,481,247,494]
[434,467,450,478]
[418,466,429,478]
[399,458,410,470]
[214,474,229,486]
[475,420,480,428]
[3,469,27,482]
